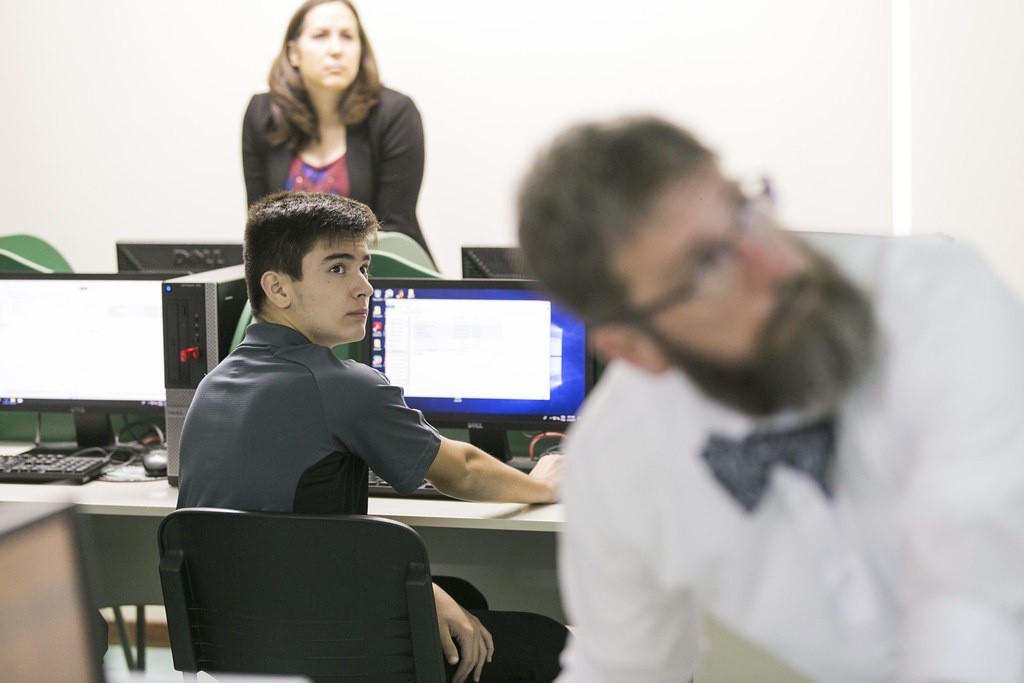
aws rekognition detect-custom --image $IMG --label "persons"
[233,0,443,276]
[172,184,566,683]
[517,108,1024,683]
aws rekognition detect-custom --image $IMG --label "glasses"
[593,173,777,325]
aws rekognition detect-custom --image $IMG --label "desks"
[0,440,566,683]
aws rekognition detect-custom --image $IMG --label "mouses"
[143,448,168,478]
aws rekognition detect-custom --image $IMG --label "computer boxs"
[161,263,250,488]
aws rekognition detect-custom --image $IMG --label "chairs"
[154,502,454,683]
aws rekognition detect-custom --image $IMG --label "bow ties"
[699,414,841,514]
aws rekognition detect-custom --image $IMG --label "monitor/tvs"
[114,243,246,272]
[1,270,195,412]
[459,247,540,278]
[366,275,597,433]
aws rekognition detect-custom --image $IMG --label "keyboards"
[1,454,112,485]
[367,466,465,501]
